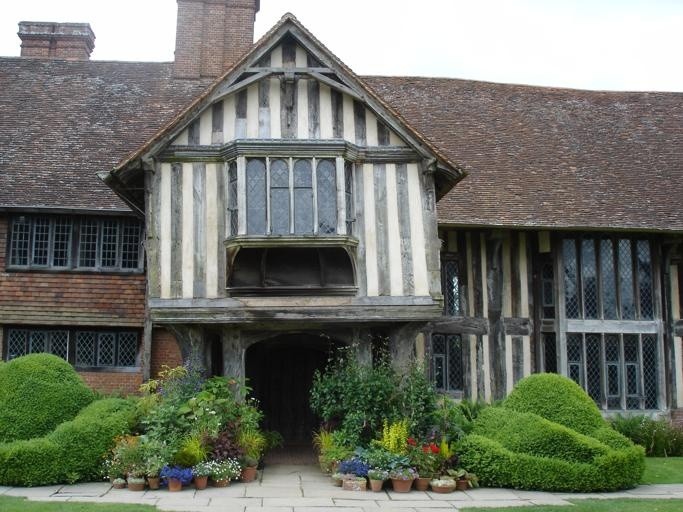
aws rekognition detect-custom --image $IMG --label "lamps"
[536,230,551,255]
[446,230,459,254]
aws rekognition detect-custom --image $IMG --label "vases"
[391,478,413,493]
[167,477,182,491]
[330,459,342,475]
[145,476,161,489]
[340,478,367,491]
[194,475,208,490]
[211,476,232,487]
[368,479,385,492]
[414,478,431,491]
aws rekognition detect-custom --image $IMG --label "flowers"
[100,348,283,483]
[334,429,442,480]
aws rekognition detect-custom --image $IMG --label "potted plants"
[311,427,343,474]
[111,477,126,488]
[333,473,357,487]
[445,468,479,491]
[127,477,146,490]
[430,475,456,493]
